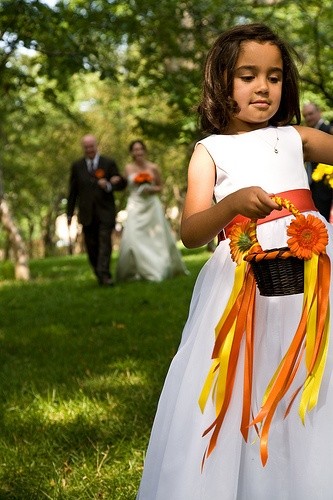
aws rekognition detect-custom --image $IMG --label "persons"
[135,21,333,500]
[111,138,191,284]
[65,134,129,286]
[301,101,333,224]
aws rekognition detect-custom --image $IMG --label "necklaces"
[239,129,279,153]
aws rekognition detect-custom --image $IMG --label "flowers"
[283,214,329,261]
[94,169,104,179]
[311,161,333,188]
[134,171,154,187]
[226,217,256,263]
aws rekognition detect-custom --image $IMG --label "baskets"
[242,197,304,297]
